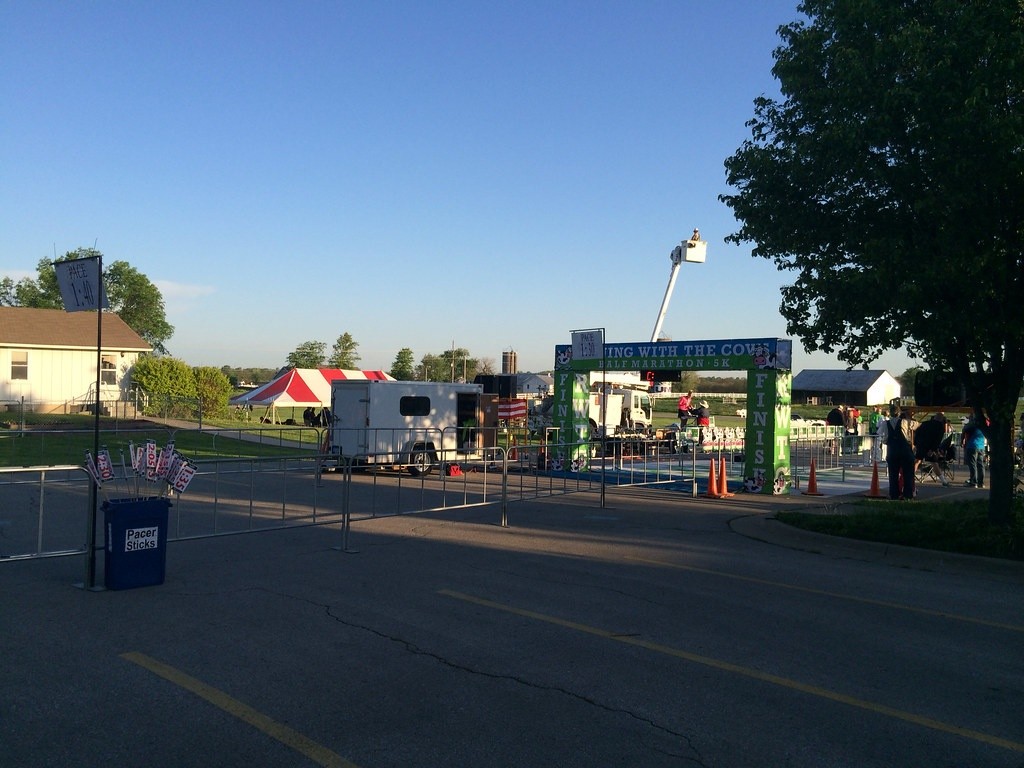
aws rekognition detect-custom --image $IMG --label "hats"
[698,399,710,408]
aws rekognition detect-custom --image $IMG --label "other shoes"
[942,481,957,488]
[963,479,985,488]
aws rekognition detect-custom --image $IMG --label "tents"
[229,368,398,425]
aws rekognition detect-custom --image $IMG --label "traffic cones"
[700,458,725,499]
[898,467,904,500]
[864,460,887,498]
[801,458,825,496]
[717,457,735,497]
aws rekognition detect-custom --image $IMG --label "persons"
[234,404,334,428]
[877,405,917,501]
[826,403,1023,489]
[677,389,693,429]
[688,399,711,445]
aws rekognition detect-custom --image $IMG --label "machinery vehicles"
[524,238,708,445]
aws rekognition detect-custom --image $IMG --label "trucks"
[327,378,498,480]
